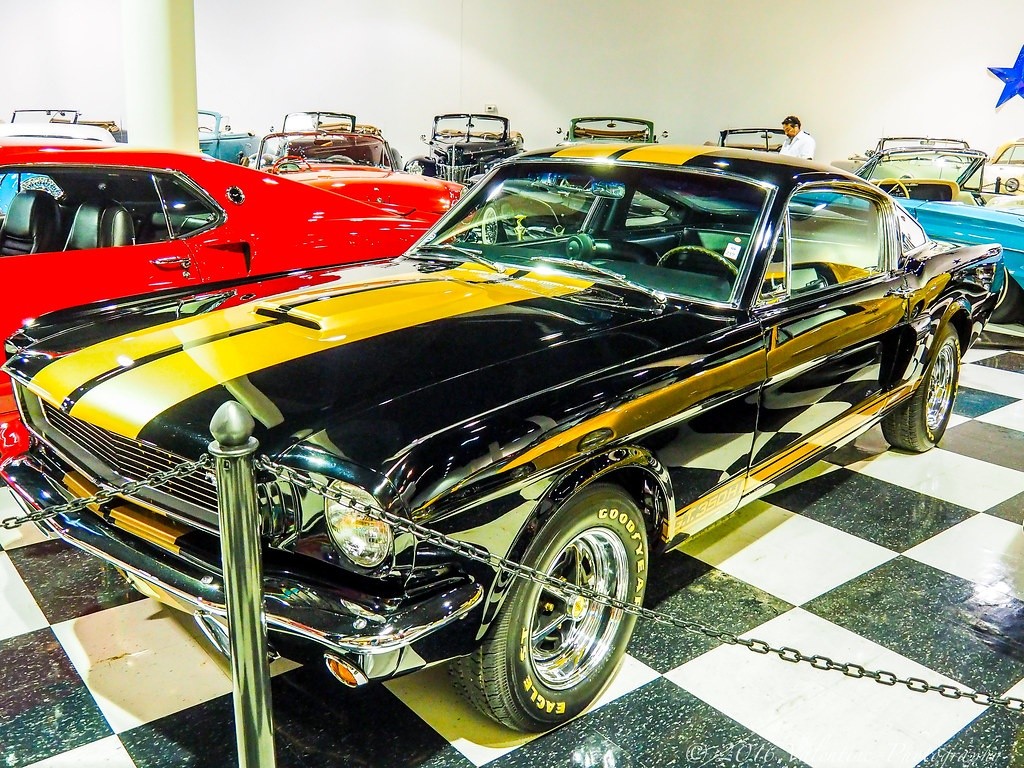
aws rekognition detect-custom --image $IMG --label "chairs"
[63,197,137,251]
[0,190,63,255]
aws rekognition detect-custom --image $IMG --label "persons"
[780,116,816,160]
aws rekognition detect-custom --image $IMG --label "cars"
[1,140,1004,736]
[0,104,1024,475]
